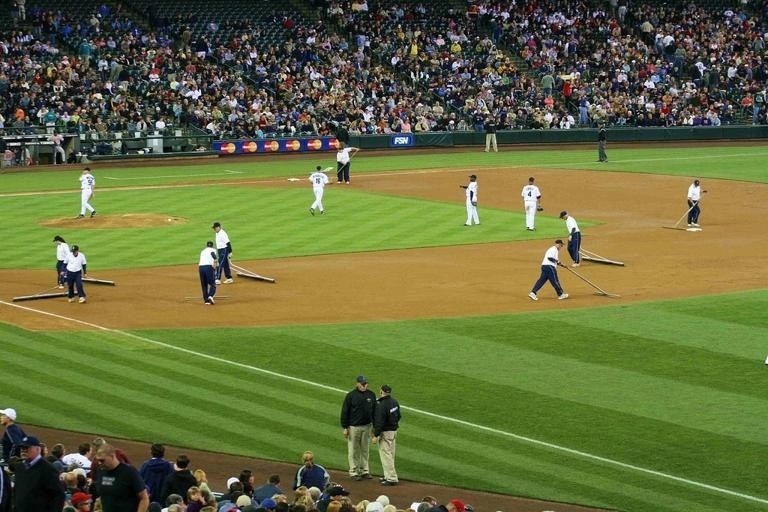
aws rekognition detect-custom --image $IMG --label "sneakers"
[350,474,363,481]
[382,481,399,486]
[223,279,233,284]
[529,292,538,301]
[379,478,386,482]
[208,296,215,304]
[557,294,568,300]
[362,473,373,479]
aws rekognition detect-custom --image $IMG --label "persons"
[340,375,402,485]
[52,236,87,303]
[336,147,360,184]
[0,1,768,166]
[198,223,232,305]
[1,408,475,512]
[77,167,96,218]
[460,175,481,226]
[309,167,328,216]
[528,240,568,301]
[559,212,581,267]
[687,180,701,227]
[521,177,541,230]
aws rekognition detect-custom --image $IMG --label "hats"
[469,175,477,178]
[237,495,252,508]
[357,376,368,385]
[213,223,220,228]
[262,498,277,510]
[452,499,464,512]
[16,437,40,448]
[72,492,92,506]
[381,385,391,393]
[227,477,240,488]
[327,484,350,496]
[410,503,421,512]
[70,245,78,252]
[559,211,567,218]
[0,408,17,421]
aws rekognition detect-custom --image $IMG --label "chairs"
[0,0,764,168]
